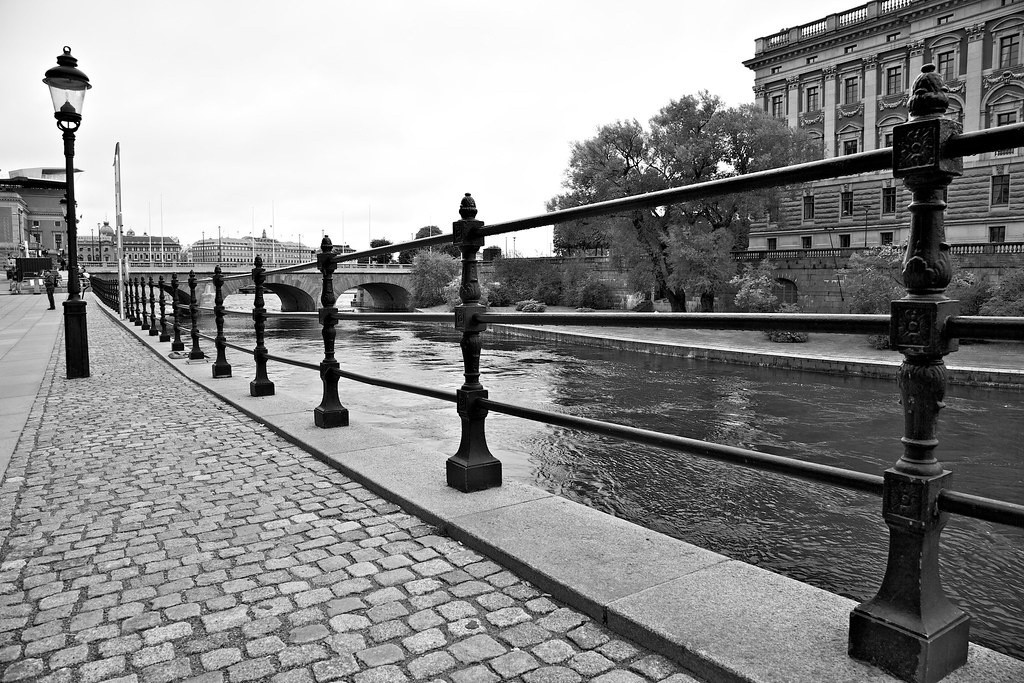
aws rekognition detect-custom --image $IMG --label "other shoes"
[47,307,55,310]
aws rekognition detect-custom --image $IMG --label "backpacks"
[48,275,58,287]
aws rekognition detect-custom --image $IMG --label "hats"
[45,270,51,273]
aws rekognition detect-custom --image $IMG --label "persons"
[44,270,56,310]
[59,258,66,271]
[81,265,86,276]
[10,267,23,295]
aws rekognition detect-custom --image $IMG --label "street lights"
[863,204,871,248]
[91,229,94,267]
[512,236,516,258]
[42,47,93,380]
[97,222,102,266]
